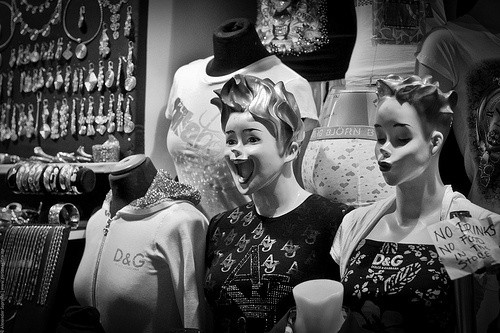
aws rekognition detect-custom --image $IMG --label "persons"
[72,154,210,333]
[330,74,500,333]
[418,1,500,214]
[204,74,356,333]
[166,17,318,224]
